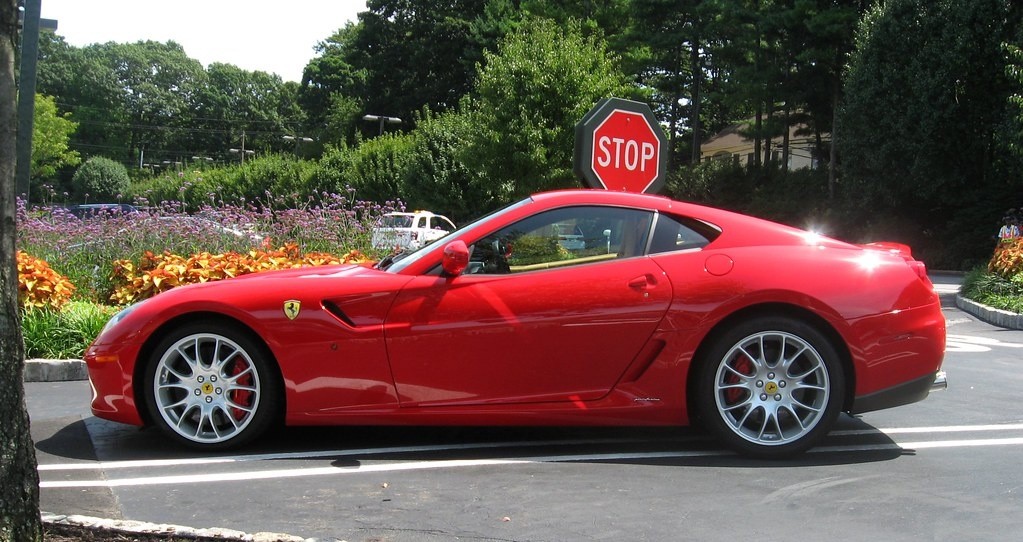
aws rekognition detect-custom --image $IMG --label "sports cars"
[82,187,951,466]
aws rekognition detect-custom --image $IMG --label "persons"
[997,216,1023,244]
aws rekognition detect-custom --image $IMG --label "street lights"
[229,130,255,167]
[162,160,182,171]
[281,131,314,161]
[143,163,160,176]
[363,114,402,137]
[192,155,214,171]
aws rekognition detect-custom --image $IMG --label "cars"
[67,203,151,226]
[551,222,586,251]
[46,215,271,289]
[370,210,457,251]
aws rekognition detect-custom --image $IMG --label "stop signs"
[571,95,669,197]
